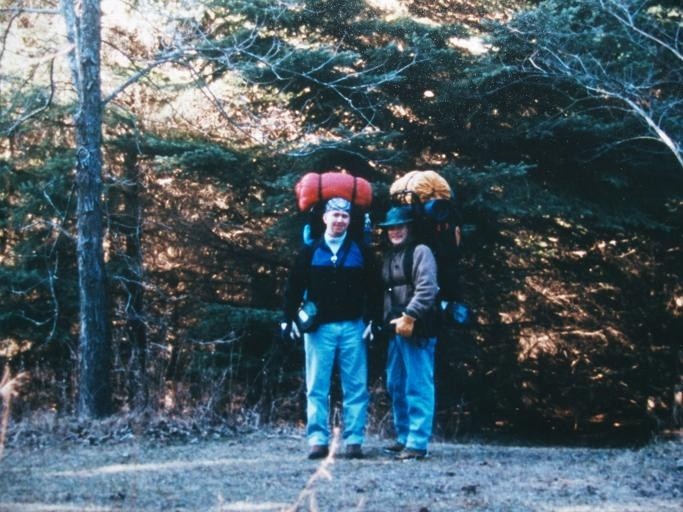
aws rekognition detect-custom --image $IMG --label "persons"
[278,198,380,460]
[377,208,440,461]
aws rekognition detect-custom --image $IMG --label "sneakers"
[386,441,403,451]
[308,446,329,459]
[346,445,361,456]
[394,448,424,460]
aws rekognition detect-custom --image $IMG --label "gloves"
[280,320,302,344]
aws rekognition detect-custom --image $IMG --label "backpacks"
[403,211,457,298]
[303,205,366,267]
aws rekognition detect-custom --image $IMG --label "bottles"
[363,213,372,242]
[441,301,467,324]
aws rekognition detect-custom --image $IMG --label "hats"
[380,205,411,227]
[324,198,352,212]
